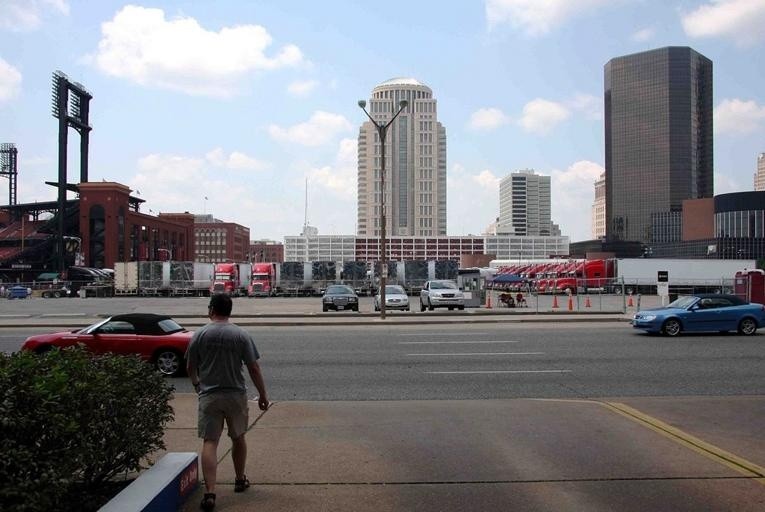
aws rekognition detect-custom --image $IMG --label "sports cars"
[18,312,198,378]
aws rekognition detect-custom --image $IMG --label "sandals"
[199,492,217,511]
[234,475,251,494]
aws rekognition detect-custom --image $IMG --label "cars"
[632,293,764,337]
[370,283,411,313]
[320,284,361,311]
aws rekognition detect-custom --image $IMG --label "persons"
[184,292,269,512]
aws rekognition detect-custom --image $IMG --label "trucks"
[481,257,758,297]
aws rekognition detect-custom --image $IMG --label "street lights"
[156,248,173,264]
[356,99,412,319]
[60,236,83,269]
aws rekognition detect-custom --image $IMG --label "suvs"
[418,280,465,311]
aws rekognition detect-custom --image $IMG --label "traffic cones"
[566,293,574,313]
[550,295,559,308]
[626,292,634,307]
[483,289,492,309]
[583,294,592,308]
